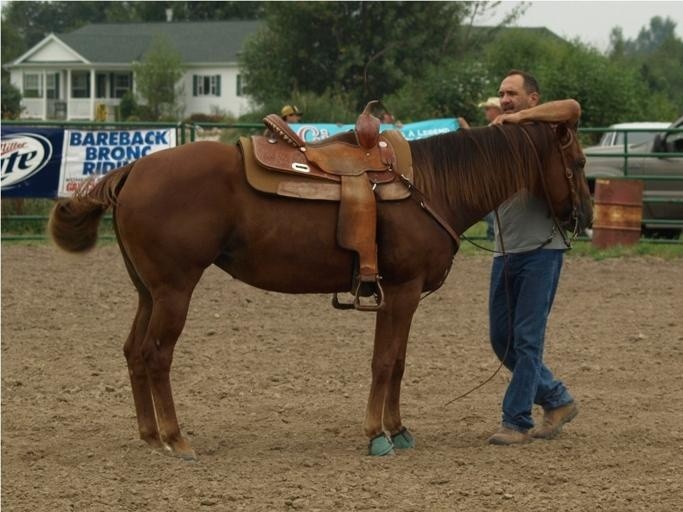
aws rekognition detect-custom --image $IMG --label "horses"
[47,118,594,460]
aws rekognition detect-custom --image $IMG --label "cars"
[601,122,672,147]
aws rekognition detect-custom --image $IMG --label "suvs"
[582,117,682,239]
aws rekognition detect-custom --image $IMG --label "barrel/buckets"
[591,177,645,250]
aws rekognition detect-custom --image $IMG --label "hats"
[478,97,500,109]
[281,105,303,118]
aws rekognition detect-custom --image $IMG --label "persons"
[486,68,582,446]
[281,104,304,123]
[457,96,502,241]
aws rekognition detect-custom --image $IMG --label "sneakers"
[531,404,578,438]
[488,424,527,445]
